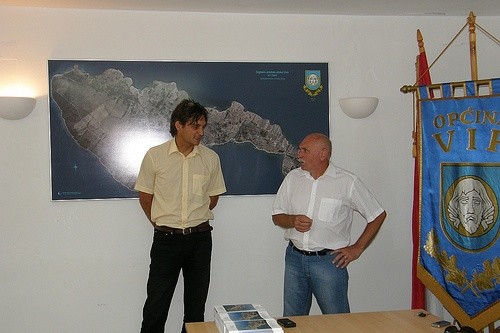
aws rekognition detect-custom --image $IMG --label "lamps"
[0,96,36,120]
[339,96,380,118]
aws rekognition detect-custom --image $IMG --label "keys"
[418,312,431,318]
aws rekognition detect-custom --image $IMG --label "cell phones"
[432,320,450,328]
[277,318,297,328]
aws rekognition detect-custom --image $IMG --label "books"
[214,304,284,333]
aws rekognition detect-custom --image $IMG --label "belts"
[288,239,334,255]
[154,220,213,235]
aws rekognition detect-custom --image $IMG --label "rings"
[344,255,347,259]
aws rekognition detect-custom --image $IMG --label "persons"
[134,98,226,333]
[270,133,387,317]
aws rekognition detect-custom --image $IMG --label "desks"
[185,309,450,333]
